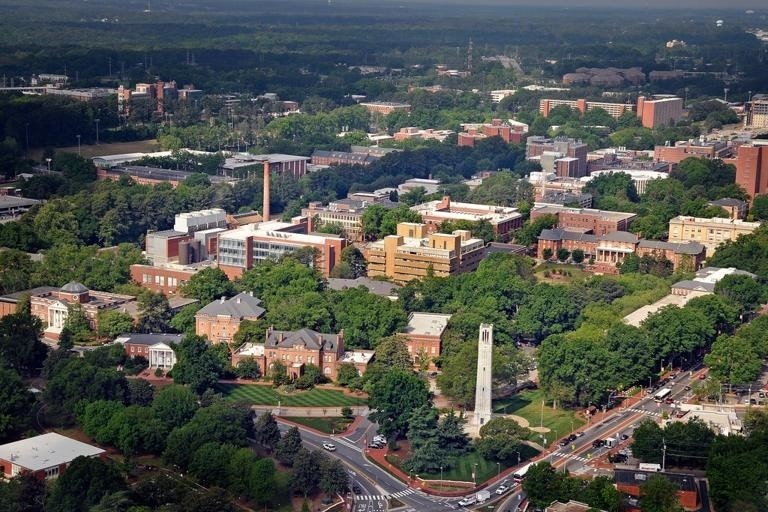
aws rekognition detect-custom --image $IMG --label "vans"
[603,437,616,449]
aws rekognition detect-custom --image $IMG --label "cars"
[759,392,767,398]
[646,373,676,394]
[665,397,673,404]
[683,385,691,391]
[368,435,388,449]
[749,398,755,405]
[559,431,584,446]
[698,374,705,379]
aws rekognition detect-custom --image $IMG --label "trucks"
[457,489,490,507]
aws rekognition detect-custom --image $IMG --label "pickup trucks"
[608,454,628,463]
[495,485,509,495]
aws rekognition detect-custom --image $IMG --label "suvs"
[592,439,603,448]
[322,444,336,452]
[352,486,359,494]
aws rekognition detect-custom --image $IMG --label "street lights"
[409,451,521,491]
[45,158,52,173]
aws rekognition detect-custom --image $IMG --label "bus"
[653,388,671,403]
[512,462,537,483]
